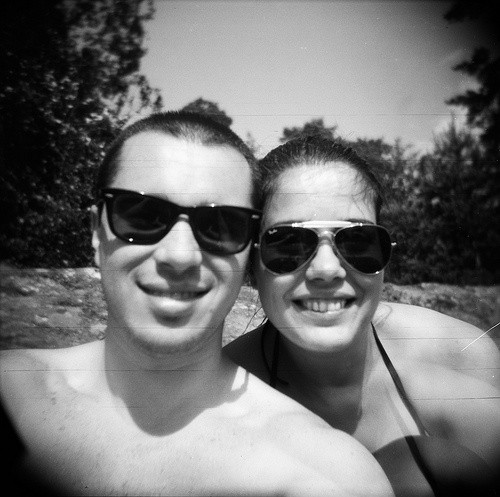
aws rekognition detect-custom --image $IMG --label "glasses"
[95,188,264,256]
[254,224,397,276]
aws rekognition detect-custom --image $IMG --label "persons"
[220,138,500,497]
[0,110,395,497]
